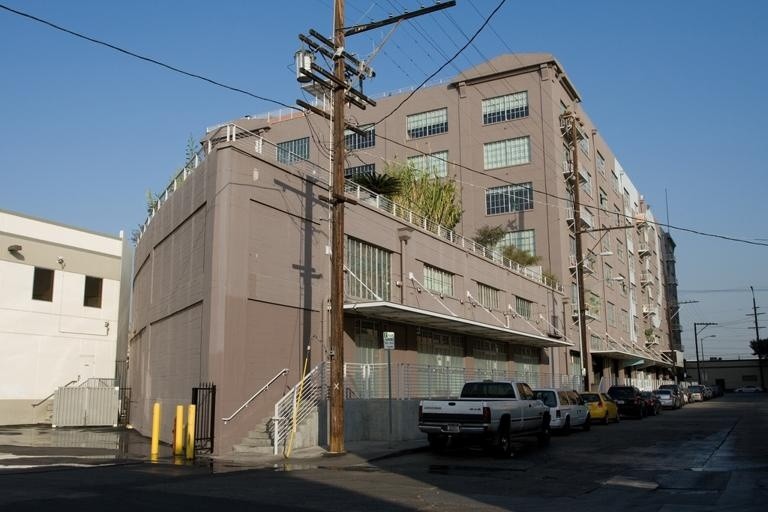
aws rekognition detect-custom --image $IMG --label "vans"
[532,387,591,436]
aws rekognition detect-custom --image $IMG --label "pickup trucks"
[418,379,549,458]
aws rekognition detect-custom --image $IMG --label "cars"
[640,391,662,416]
[651,384,724,410]
[580,392,621,425]
[735,385,761,393]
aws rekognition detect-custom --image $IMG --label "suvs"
[607,386,643,419]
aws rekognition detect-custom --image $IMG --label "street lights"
[701,334,715,383]
[576,251,614,388]
